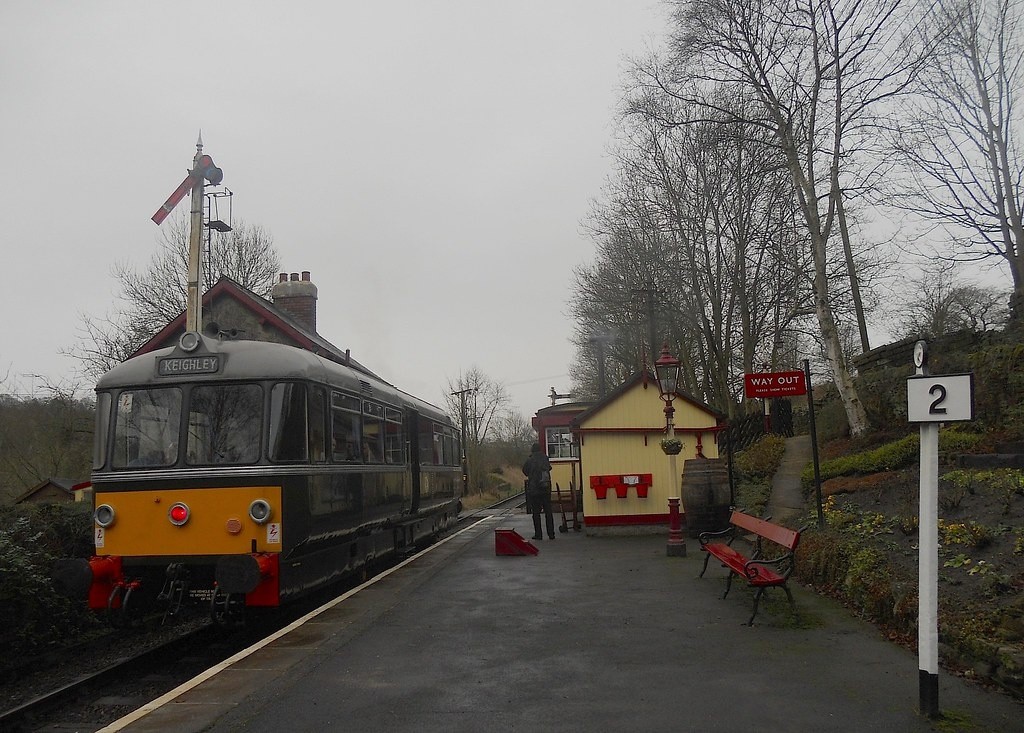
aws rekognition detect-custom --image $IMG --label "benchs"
[696,508,810,630]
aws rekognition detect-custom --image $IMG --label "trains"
[71,127,466,638]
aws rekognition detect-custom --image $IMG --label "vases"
[662,448,682,456]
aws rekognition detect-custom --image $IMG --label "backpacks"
[529,454,550,491]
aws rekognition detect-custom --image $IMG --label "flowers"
[659,437,687,451]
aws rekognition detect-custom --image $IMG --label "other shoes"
[549,536,555,539]
[532,536,542,539]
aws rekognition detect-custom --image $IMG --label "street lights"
[652,343,686,556]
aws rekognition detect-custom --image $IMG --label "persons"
[319,439,374,461]
[127,435,192,466]
[522,443,555,539]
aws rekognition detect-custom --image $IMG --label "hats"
[531,443,541,453]
